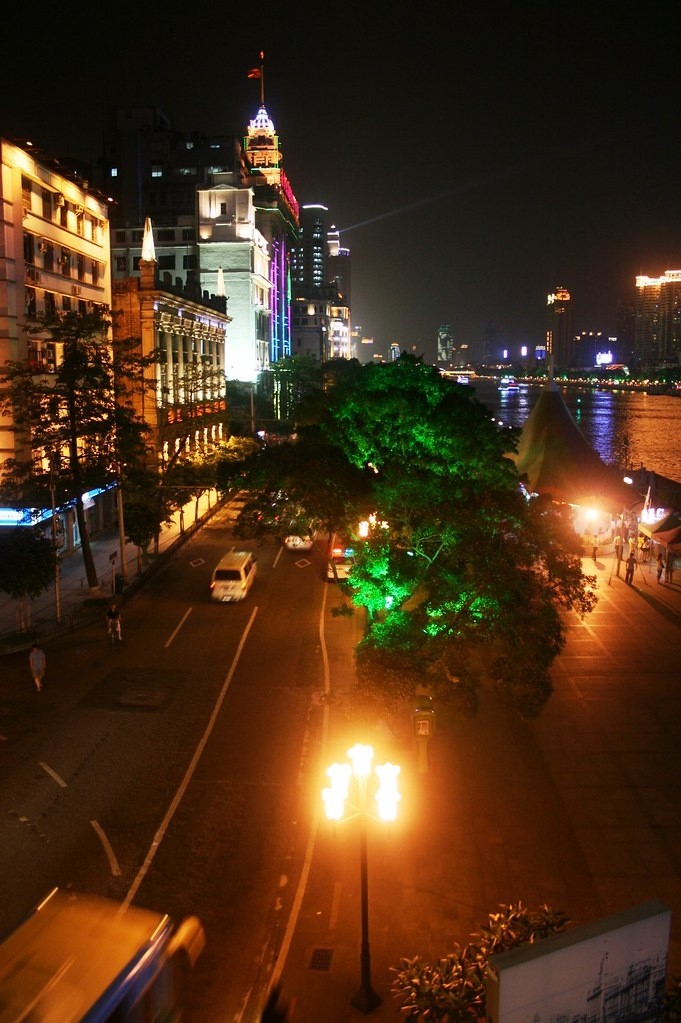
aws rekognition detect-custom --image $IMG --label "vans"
[210,546,258,602]
[458,377,469,384]
[285,515,322,554]
[327,529,357,579]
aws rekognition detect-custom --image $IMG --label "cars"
[260,417,302,452]
[256,501,285,529]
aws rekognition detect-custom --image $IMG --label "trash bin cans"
[111,572,124,595]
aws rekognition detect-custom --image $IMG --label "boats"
[497,376,520,390]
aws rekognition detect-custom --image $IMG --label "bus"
[0,884,206,1023]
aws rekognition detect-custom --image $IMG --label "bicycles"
[106,618,120,646]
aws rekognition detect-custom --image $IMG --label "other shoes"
[117,636,122,640]
[37,688,40,692]
[107,630,112,634]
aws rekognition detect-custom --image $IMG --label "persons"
[628,534,649,562]
[625,552,638,586]
[664,553,676,583]
[28,643,47,692]
[105,603,123,641]
[614,533,625,561]
[591,534,599,561]
[656,553,665,585]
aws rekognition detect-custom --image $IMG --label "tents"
[638,512,681,557]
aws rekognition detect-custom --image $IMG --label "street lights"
[320,738,402,1018]
